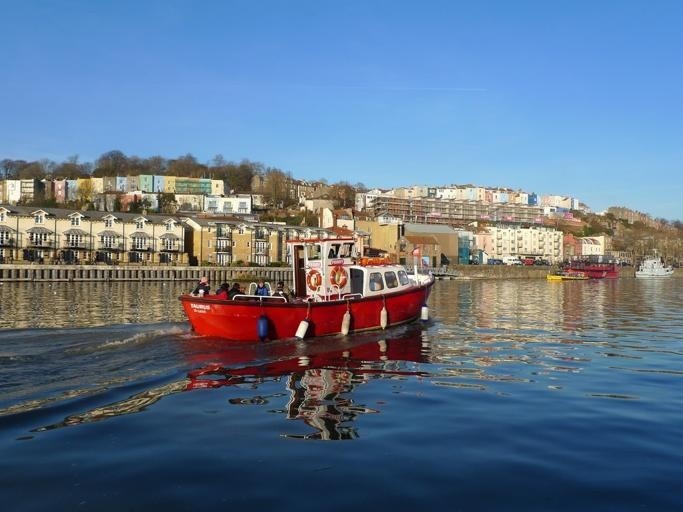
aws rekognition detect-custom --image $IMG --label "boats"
[179,231,435,344]
[546,255,676,280]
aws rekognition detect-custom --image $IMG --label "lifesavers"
[307,270,321,291]
[330,266,348,288]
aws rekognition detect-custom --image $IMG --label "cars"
[484,254,545,266]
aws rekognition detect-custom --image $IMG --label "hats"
[199,277,208,285]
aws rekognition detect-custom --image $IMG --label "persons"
[277,281,293,302]
[191,276,210,296]
[228,283,249,301]
[215,282,228,300]
[253,280,268,300]
